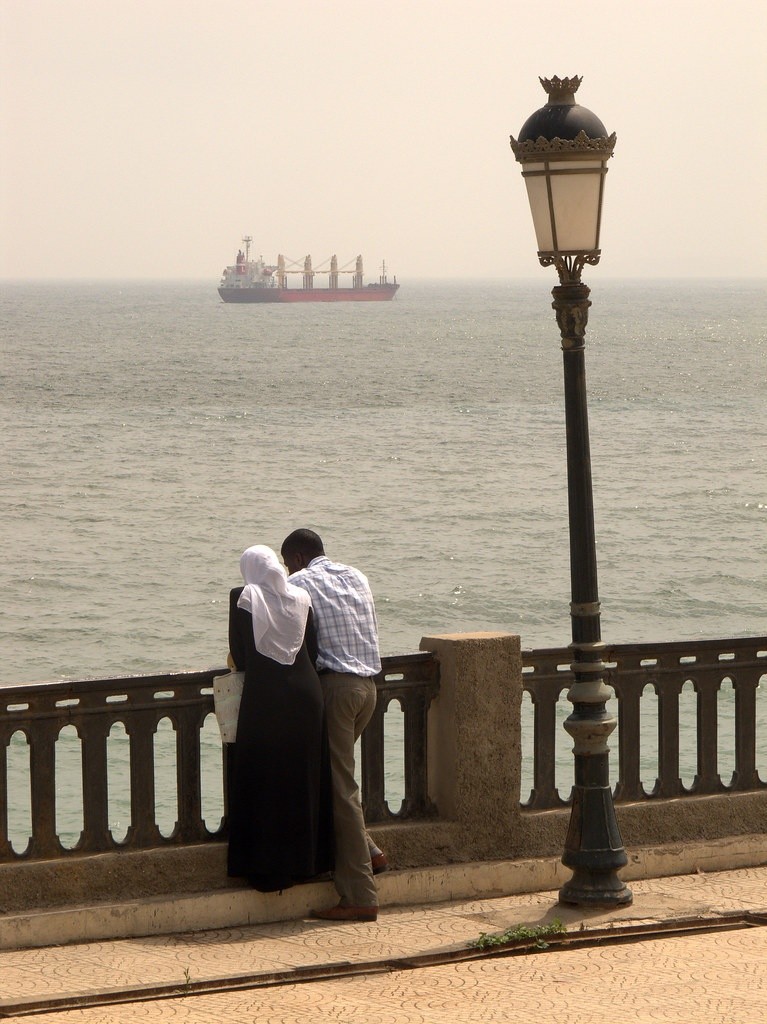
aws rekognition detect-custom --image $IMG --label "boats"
[217,236,399,303]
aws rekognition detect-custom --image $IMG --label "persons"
[226,544,333,894]
[280,528,390,921]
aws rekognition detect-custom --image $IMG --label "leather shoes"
[372,853,386,874]
[310,905,378,921]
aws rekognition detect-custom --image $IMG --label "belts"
[317,668,335,676]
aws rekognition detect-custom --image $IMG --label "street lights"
[510,75,638,908]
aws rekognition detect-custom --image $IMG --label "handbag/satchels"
[213,663,245,744]
